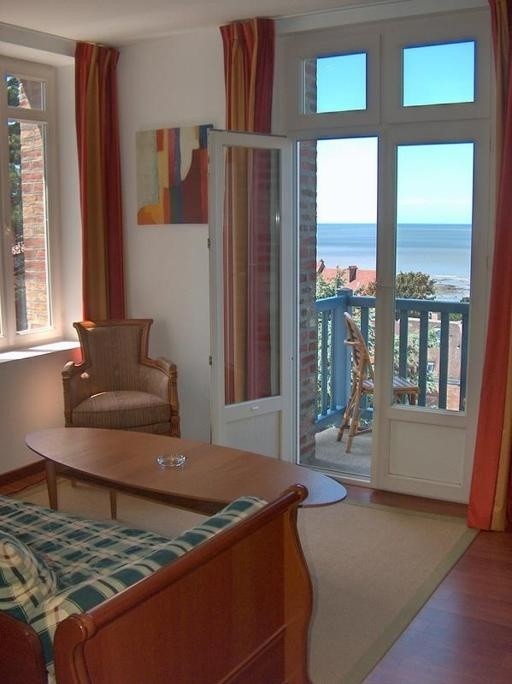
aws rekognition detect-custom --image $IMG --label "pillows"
[0,529,63,624]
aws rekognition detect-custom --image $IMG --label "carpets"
[5,477,482,683]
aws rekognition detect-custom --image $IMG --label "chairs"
[0,484,313,684]
[61,318,181,439]
[337,312,421,455]
